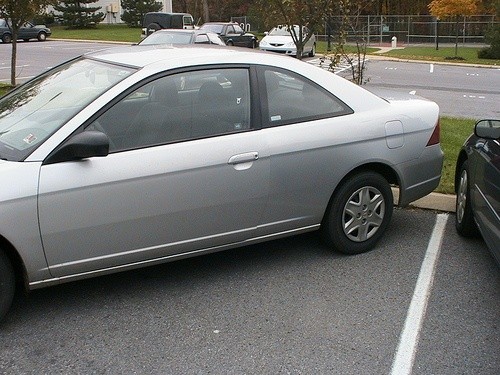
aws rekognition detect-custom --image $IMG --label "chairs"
[187,80,233,138]
[119,78,187,150]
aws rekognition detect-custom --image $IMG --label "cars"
[136,28,225,48]
[259,23,317,58]
[201,21,259,49]
[453,117,500,268]
[0,43,445,318]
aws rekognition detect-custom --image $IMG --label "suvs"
[0,16,52,43]
[142,11,200,43]
